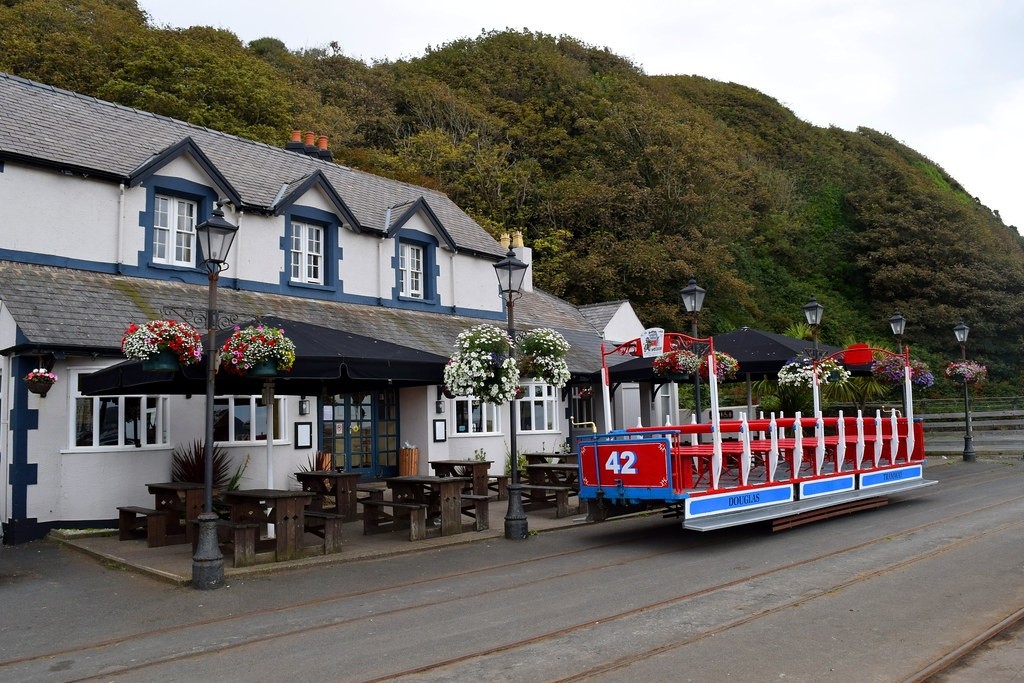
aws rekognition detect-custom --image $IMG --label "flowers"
[23,368,57,384]
[219,323,296,375]
[945,361,985,386]
[653,350,700,376]
[871,355,935,388]
[777,357,849,389]
[699,352,739,378]
[443,326,568,405]
[579,386,594,398]
[121,319,204,366]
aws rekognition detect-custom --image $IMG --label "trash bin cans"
[317,452,331,486]
[400,448,418,476]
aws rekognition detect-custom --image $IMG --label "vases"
[827,370,839,383]
[703,375,724,386]
[967,380,976,384]
[248,356,280,376]
[26,380,54,394]
[581,393,591,400]
[951,373,966,382]
[136,347,180,376]
[516,393,525,399]
[671,372,690,383]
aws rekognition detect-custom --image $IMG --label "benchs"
[116,474,584,567]
[624,407,909,490]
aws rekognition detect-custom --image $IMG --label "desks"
[427,460,496,503]
[523,463,579,509]
[525,452,578,487]
[227,489,316,562]
[294,470,364,522]
[143,482,222,544]
[381,475,473,537]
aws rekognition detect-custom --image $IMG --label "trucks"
[679,404,764,446]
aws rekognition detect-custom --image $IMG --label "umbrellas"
[590,326,875,467]
[82,316,452,539]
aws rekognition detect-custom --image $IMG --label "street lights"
[952,316,977,463]
[492,231,529,540]
[679,272,707,445]
[888,307,907,417]
[194,196,240,590]
[802,292,825,412]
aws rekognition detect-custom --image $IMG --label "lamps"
[435,401,446,413]
[298,400,310,415]
